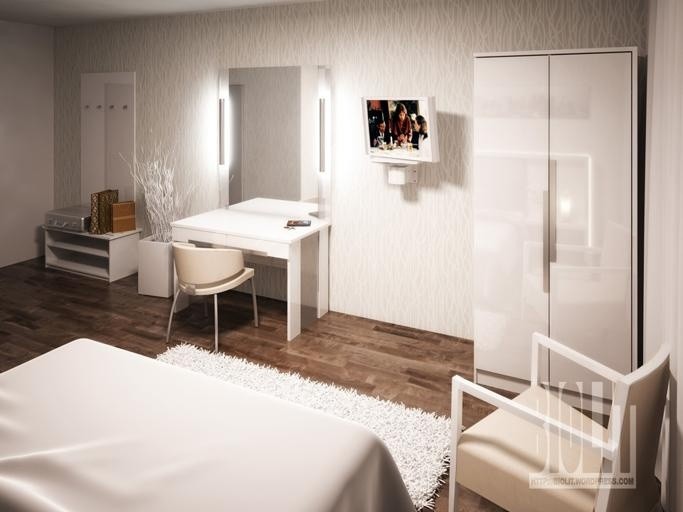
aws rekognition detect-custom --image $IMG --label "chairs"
[165,242,258,352]
[444,333,671,512]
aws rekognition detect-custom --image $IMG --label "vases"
[136,233,171,299]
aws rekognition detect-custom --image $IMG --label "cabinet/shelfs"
[38,225,142,284]
[467,44,639,415]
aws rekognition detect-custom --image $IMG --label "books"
[110,200,136,233]
[99,188,120,236]
[89,189,101,233]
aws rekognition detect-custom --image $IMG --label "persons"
[391,101,411,145]
[410,114,428,150]
[369,118,391,148]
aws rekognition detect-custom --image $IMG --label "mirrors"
[215,63,325,217]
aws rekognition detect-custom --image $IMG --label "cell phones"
[287,220,311,225]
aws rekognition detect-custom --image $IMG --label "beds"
[0,334,391,511]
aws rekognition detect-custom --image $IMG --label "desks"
[228,196,317,211]
[168,207,332,341]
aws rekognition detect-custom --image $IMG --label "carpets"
[143,342,467,510]
